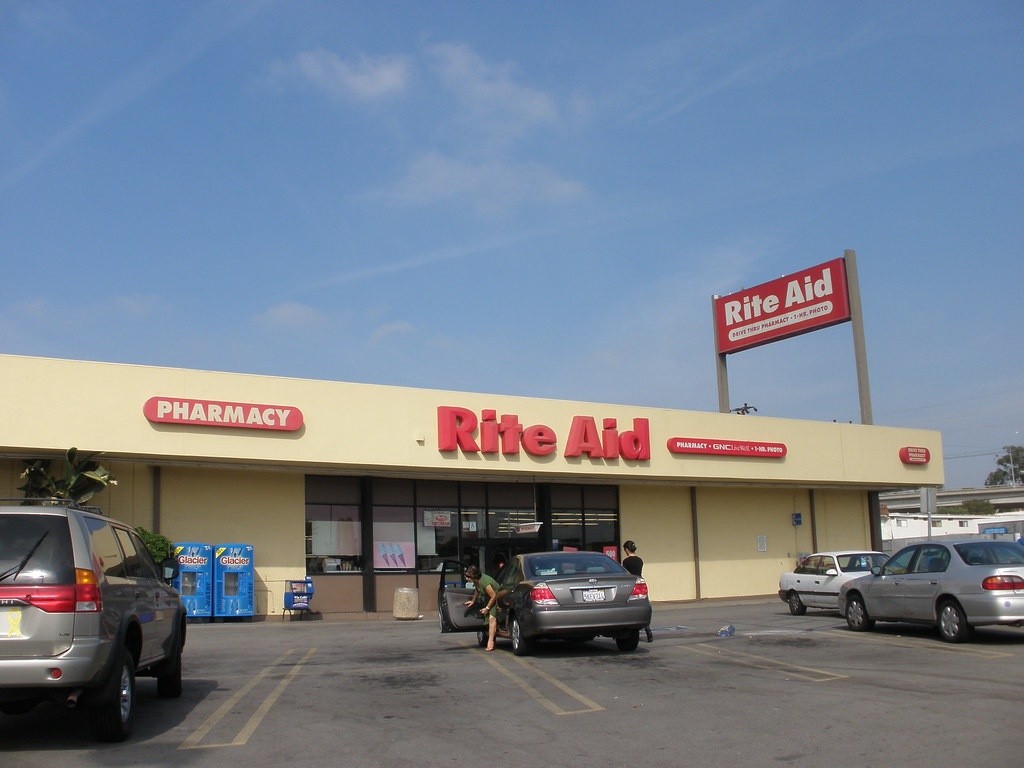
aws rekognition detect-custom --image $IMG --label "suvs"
[1,496,188,743]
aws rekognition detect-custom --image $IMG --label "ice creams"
[379,543,407,567]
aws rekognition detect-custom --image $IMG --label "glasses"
[465,575,471,578]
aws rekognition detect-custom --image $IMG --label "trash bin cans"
[392,587,419,620]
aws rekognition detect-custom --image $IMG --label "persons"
[463,565,501,651]
[623,540,654,643]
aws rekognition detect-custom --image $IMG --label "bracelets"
[486,607,490,610]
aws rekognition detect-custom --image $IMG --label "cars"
[438,550,653,656]
[418,538,530,583]
[839,534,1024,644]
[778,550,904,617]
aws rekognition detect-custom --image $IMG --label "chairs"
[928,559,947,572]
[535,562,607,573]
[825,563,836,570]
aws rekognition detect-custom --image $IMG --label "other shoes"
[647,631,652,642]
[485,642,494,650]
